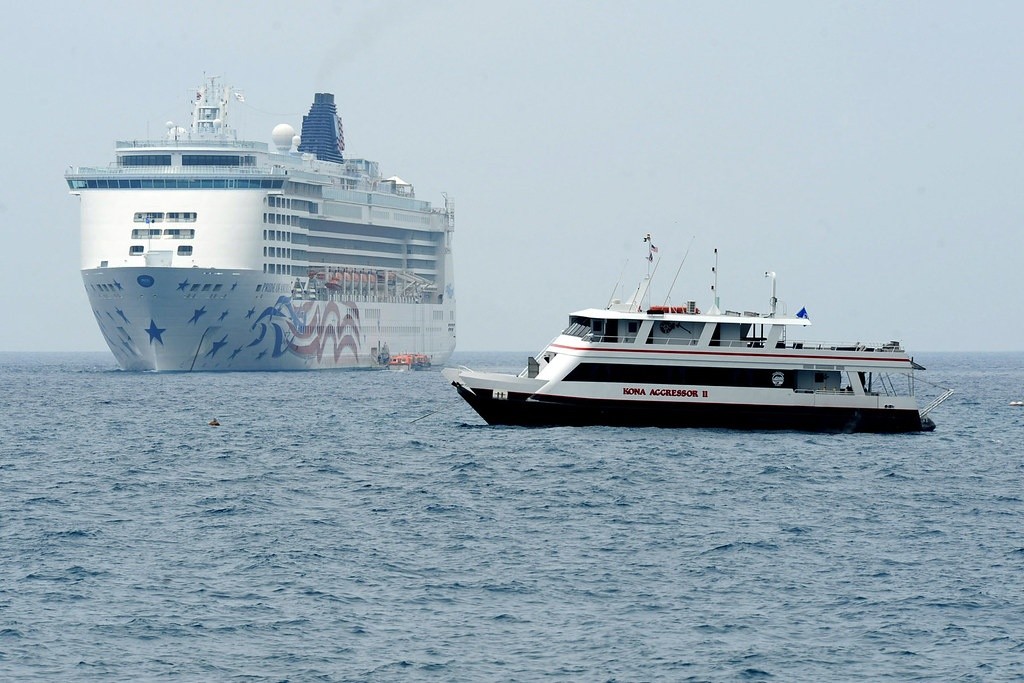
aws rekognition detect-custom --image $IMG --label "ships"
[62,69,457,372]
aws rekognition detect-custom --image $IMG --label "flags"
[146,218,149,224]
[196,92,201,102]
[235,92,244,102]
[650,244,658,252]
[794,306,810,328]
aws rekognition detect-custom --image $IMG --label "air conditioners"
[686,299,696,313]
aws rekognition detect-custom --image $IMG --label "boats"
[387,353,432,372]
[440,232,954,433]
[362,274,368,288]
[346,273,352,289]
[370,275,376,290]
[324,273,343,290]
[308,271,325,279]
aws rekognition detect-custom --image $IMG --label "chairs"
[857,345,867,351]
